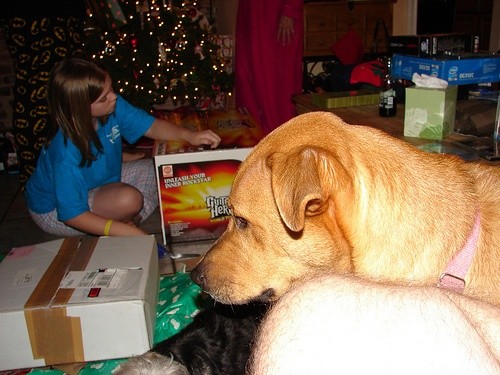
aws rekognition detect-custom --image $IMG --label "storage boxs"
[0,234,158,371]
[391,54,500,85]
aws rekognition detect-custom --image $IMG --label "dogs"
[190,110,500,313]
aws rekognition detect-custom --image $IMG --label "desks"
[292,92,500,165]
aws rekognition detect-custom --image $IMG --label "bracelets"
[103,219,113,236]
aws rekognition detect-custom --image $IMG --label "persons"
[22,54,222,237]
[246,269,500,375]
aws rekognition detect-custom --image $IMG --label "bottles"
[379,56,398,117]
